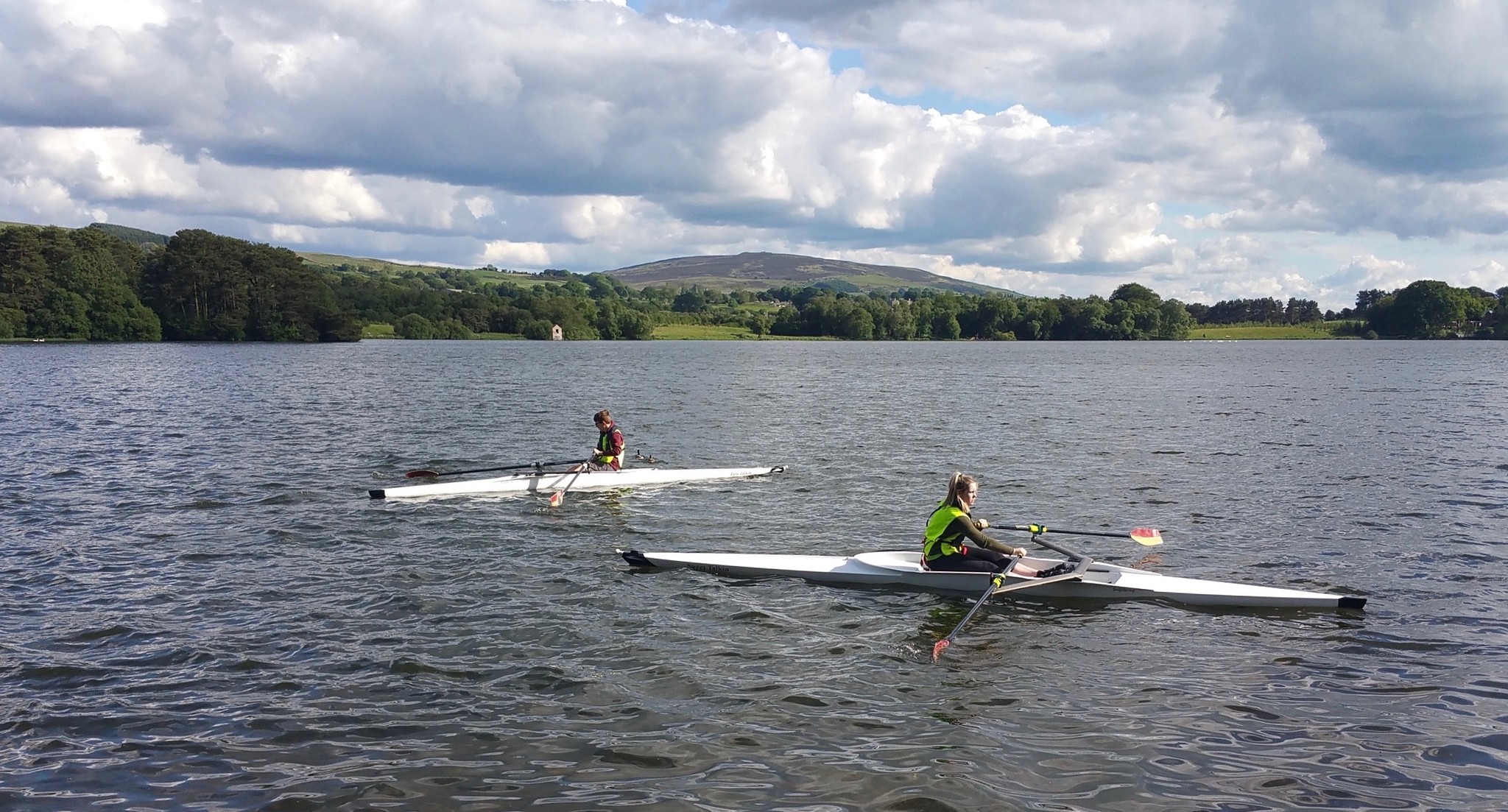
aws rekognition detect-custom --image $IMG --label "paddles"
[977,522,1164,546]
[550,452,596,505]
[406,458,598,477]
[933,552,1023,660]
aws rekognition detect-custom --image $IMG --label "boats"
[368,464,791,501]
[615,525,1370,613]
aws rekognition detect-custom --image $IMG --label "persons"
[568,411,625,471]
[920,473,1075,577]
